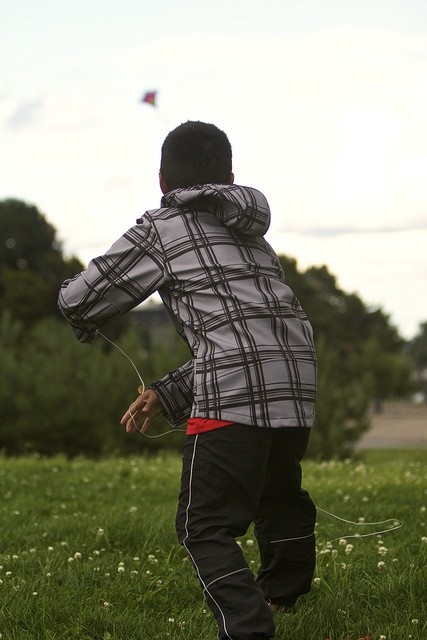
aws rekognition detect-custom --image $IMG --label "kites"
[144,91,155,106]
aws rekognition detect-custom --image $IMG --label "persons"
[58,121,318,640]
